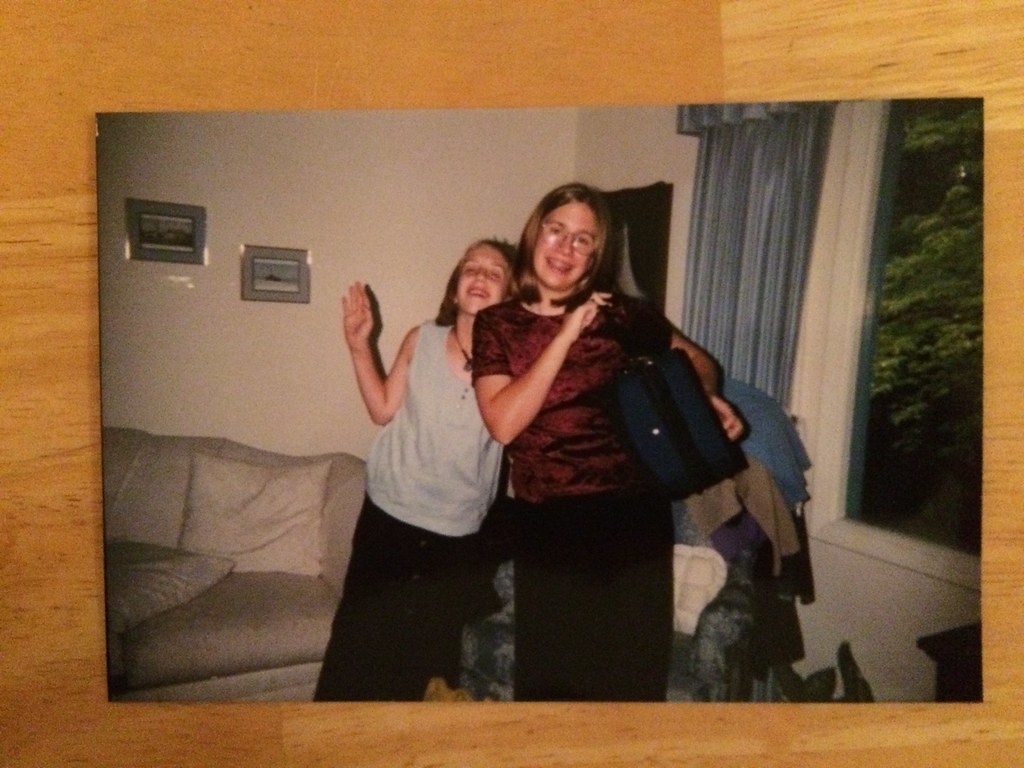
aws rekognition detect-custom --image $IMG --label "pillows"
[100,537,233,634]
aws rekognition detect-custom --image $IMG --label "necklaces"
[453,330,474,370]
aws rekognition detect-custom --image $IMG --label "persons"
[471,184,745,702]
[308,241,538,702]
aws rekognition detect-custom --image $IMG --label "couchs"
[98,420,365,703]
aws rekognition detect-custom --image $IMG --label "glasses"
[542,221,595,256]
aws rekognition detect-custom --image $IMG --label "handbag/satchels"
[618,346,750,510]
